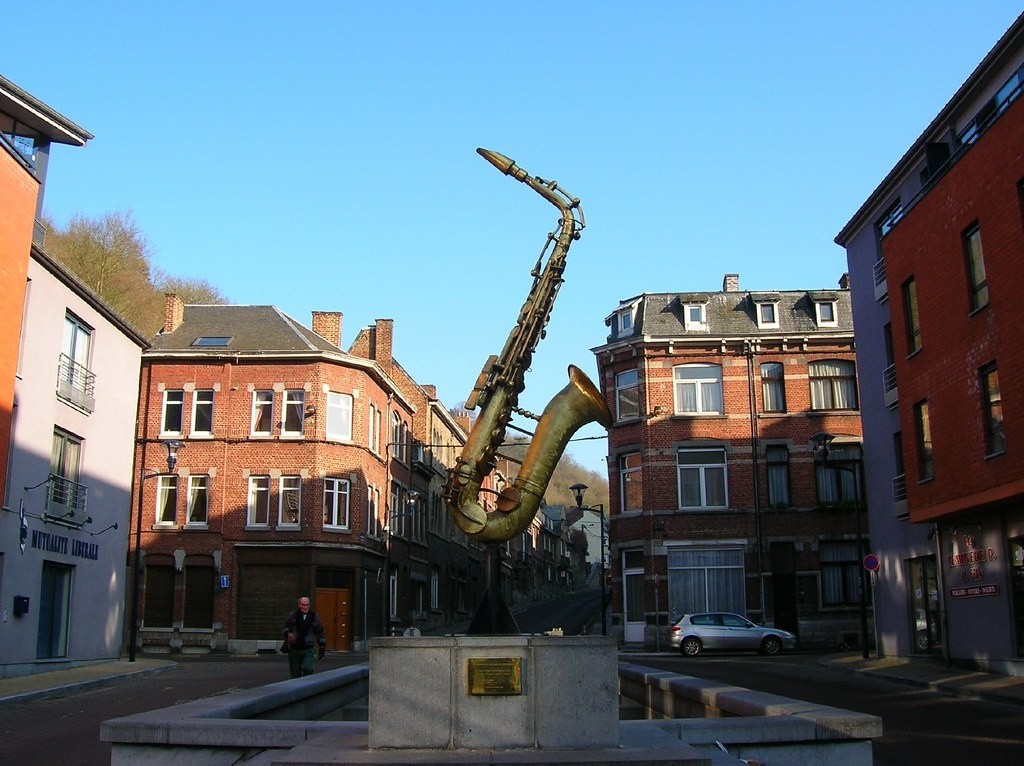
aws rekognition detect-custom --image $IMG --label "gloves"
[318,648,326,661]
[284,629,289,639]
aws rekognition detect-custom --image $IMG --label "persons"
[281,597,326,679]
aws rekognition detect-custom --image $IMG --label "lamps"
[68,516,92,530]
[24,472,53,491]
[90,523,118,536]
[44,510,74,524]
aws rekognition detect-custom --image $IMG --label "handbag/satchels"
[280,638,289,654]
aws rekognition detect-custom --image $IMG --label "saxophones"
[440,146,613,546]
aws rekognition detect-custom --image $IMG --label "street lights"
[385,490,421,637]
[569,482,607,636]
[809,432,870,660]
[127,439,186,662]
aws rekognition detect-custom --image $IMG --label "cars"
[669,612,795,657]
[915,608,937,654]
